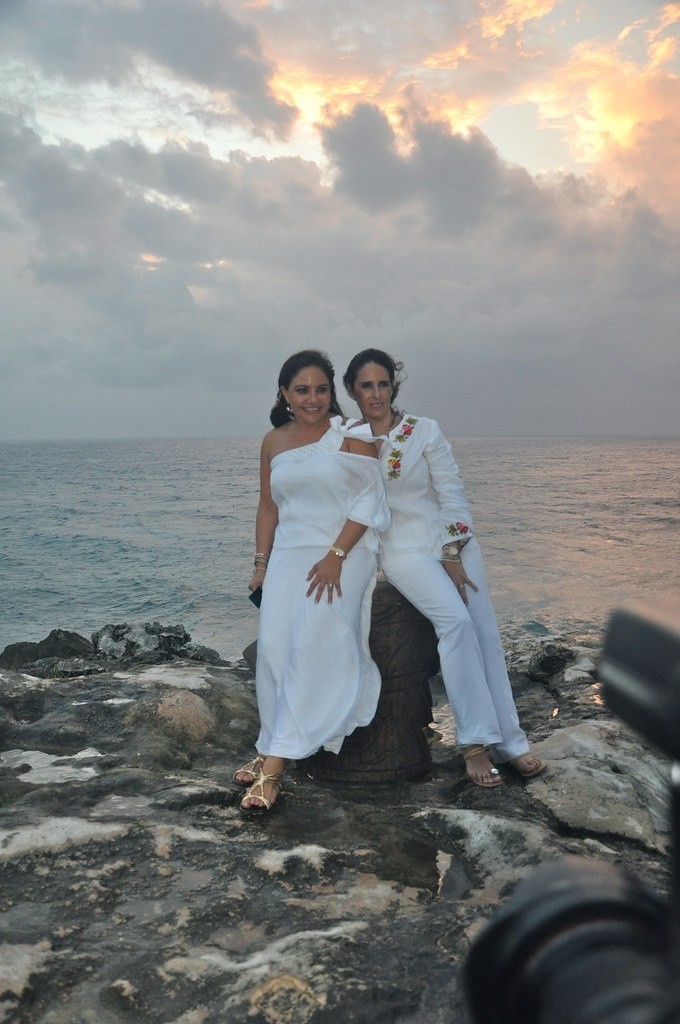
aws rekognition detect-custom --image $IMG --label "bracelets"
[440,545,461,563]
[252,553,269,574]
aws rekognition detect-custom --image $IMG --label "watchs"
[331,544,347,560]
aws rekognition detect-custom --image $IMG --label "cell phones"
[248,587,263,608]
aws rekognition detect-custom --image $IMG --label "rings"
[459,584,463,588]
[328,584,332,586]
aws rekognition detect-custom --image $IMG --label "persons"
[342,348,547,786]
[232,350,391,814]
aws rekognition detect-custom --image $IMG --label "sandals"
[231,754,265,786]
[238,769,282,816]
[512,753,548,778]
[461,746,503,788]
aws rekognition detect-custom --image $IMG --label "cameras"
[458,599,680,1024]
